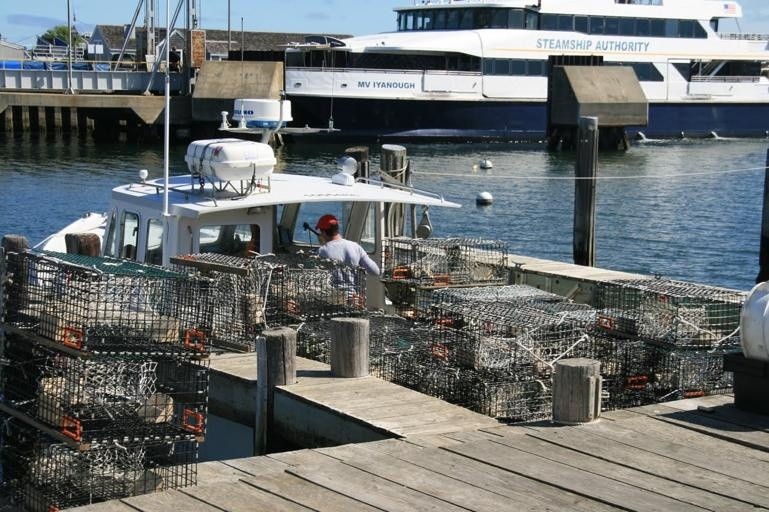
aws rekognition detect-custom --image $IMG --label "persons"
[168,46,180,72]
[311,214,383,295]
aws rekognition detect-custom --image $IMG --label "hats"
[315,214,338,231]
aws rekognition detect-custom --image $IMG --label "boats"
[227,0,769,135]
[33,97,513,356]
